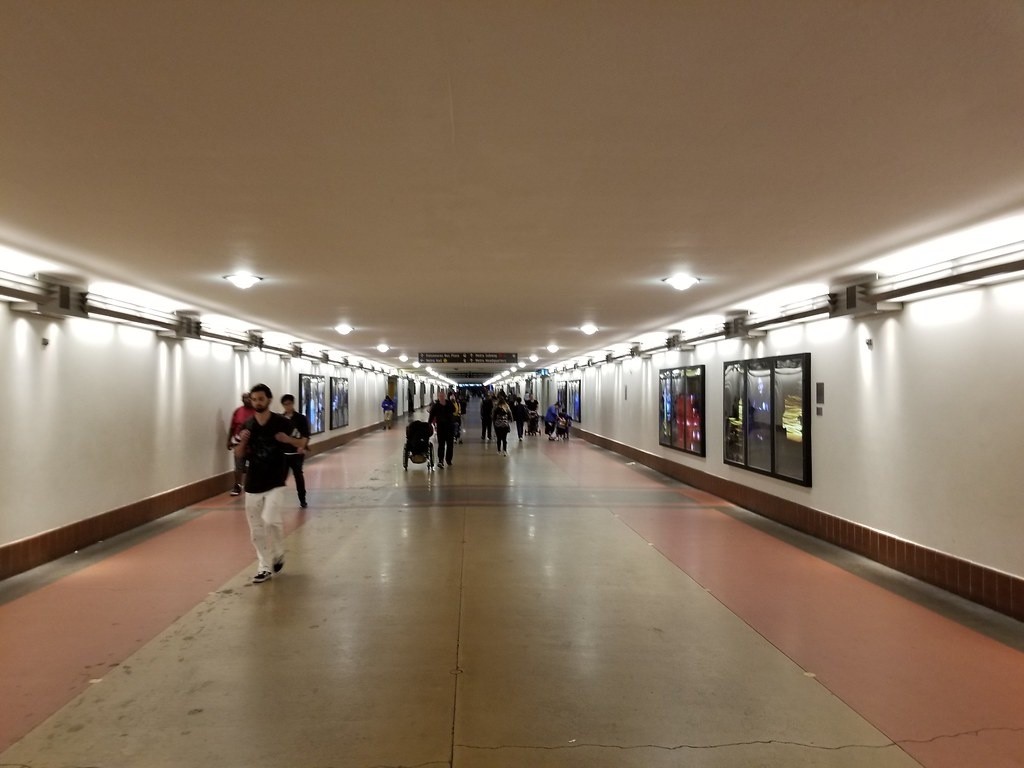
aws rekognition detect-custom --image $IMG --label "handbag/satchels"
[566,414,572,427]
[523,403,531,423]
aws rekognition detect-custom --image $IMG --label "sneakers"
[252,571,272,582]
[273,559,284,573]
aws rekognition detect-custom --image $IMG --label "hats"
[450,395,455,401]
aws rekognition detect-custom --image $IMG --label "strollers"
[453,414,462,442]
[402,420,434,469]
[525,410,541,436]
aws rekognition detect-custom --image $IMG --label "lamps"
[0,270,52,303]
[741,293,836,333]
[677,323,730,346]
[859,239,1024,303]
[82,291,182,332]
[579,325,599,335]
[198,322,457,387]
[662,276,700,290]
[222,275,263,290]
[483,339,671,388]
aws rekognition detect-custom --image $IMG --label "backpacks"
[559,417,566,429]
[495,413,509,428]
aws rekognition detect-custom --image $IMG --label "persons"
[226,393,258,496]
[428,386,541,468]
[381,394,396,430]
[239,383,308,583]
[544,401,573,443]
[280,394,311,508]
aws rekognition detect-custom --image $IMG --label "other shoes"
[503,451,507,457]
[230,483,241,495]
[519,437,523,441]
[383,426,386,430]
[549,437,555,441]
[497,451,502,456]
[487,436,492,440]
[437,461,444,469]
[481,436,485,440]
[299,497,307,508]
[445,457,452,466]
[388,426,391,430]
